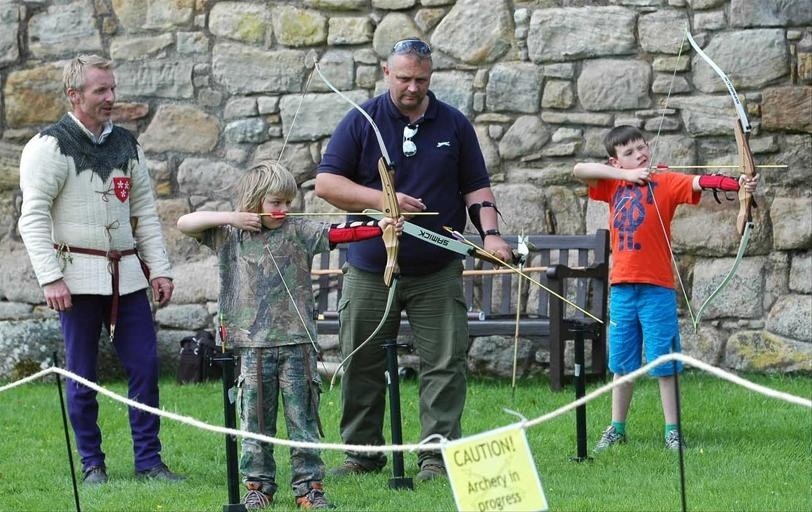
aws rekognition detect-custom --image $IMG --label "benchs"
[308,227,612,392]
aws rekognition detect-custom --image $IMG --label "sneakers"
[415,459,447,485]
[81,465,110,486]
[330,461,372,477]
[140,464,186,484]
[597,426,626,449]
[241,481,274,511]
[295,481,335,510]
[664,430,687,449]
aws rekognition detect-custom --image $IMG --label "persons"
[572,124,760,455]
[314,34,514,483]
[175,161,405,512]
[16,52,188,485]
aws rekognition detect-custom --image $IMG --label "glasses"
[391,38,432,58]
